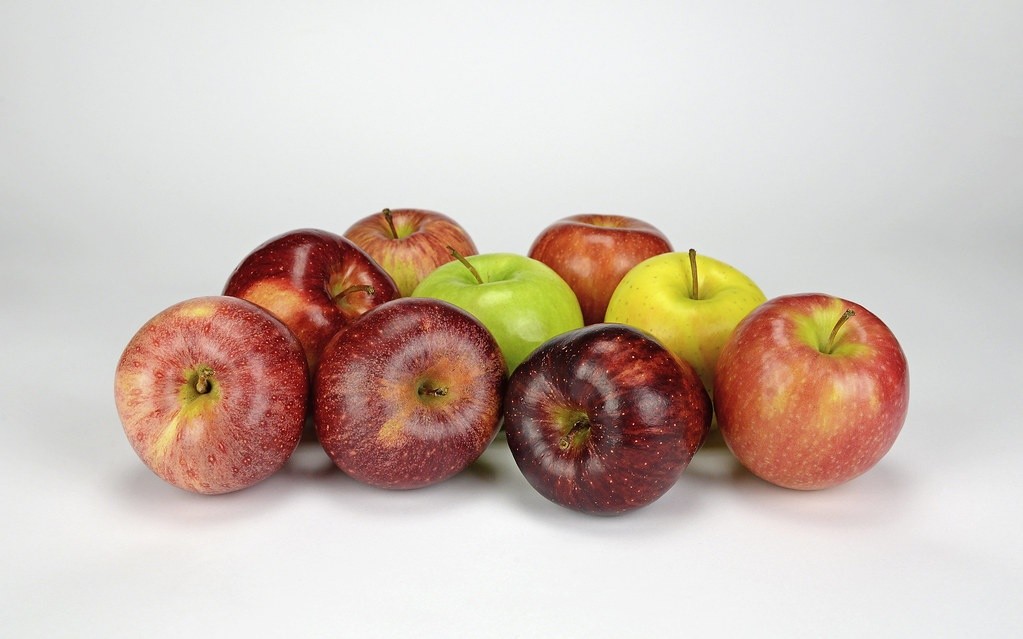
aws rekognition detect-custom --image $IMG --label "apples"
[311,297,509,489]
[712,292,910,492]
[343,208,477,299]
[410,244,585,374]
[221,227,401,410]
[603,248,768,397]
[527,212,675,327]
[505,322,714,515]
[113,295,308,495]
